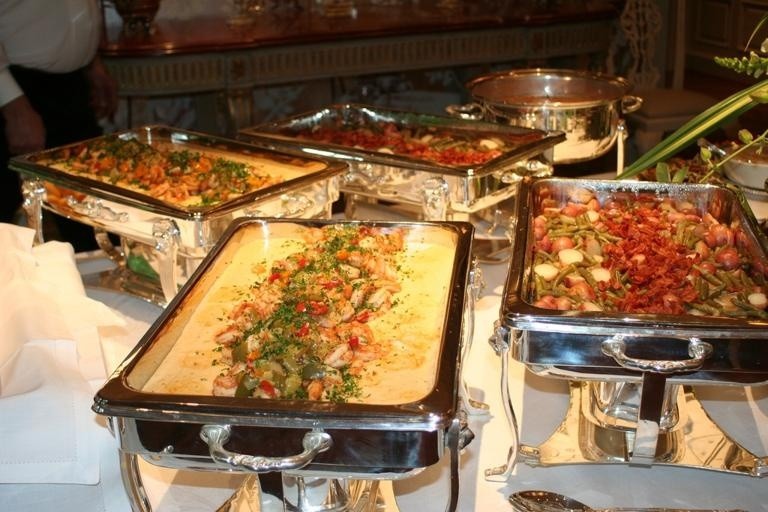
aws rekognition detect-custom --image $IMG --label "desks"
[62,150,764,512]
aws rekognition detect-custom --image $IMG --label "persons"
[0,1,118,221]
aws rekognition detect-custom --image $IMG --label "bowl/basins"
[719,140,768,190]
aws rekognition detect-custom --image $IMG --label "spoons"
[510,489,749,512]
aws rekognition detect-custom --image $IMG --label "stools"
[618,83,743,165]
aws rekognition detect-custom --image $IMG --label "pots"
[445,68,644,166]
